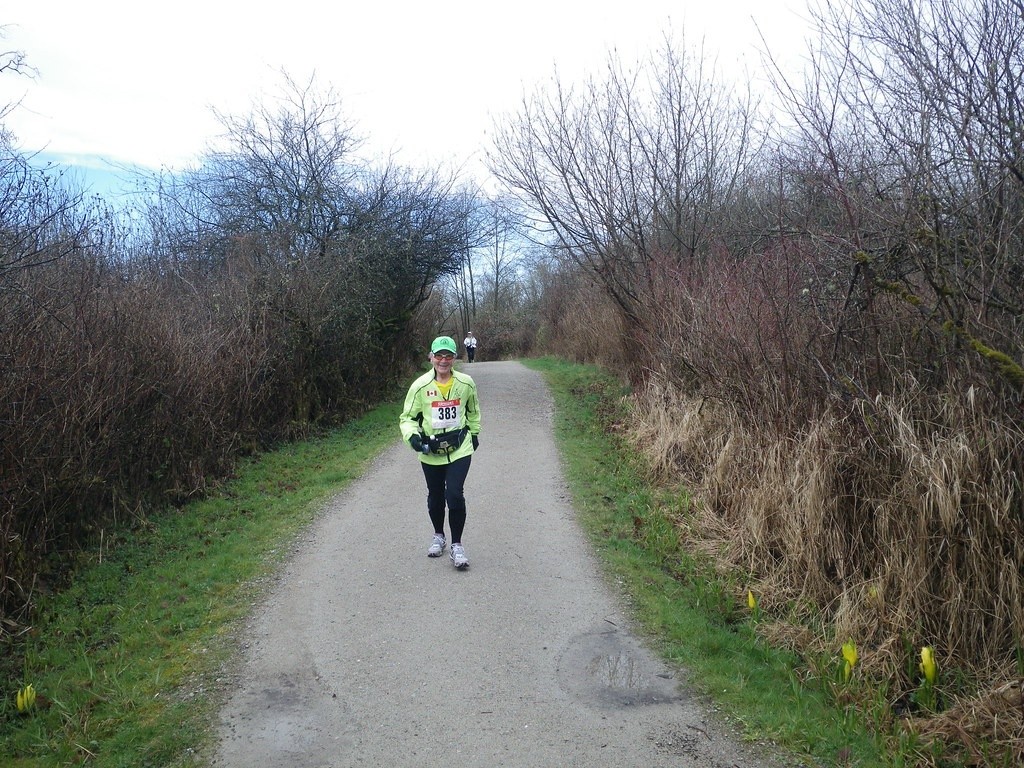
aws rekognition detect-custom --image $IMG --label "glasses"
[433,353,455,360]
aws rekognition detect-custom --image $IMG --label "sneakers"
[428,533,447,557]
[449,543,469,568]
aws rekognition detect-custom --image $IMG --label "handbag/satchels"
[421,425,470,455]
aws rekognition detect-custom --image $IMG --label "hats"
[468,332,472,334]
[431,336,456,355]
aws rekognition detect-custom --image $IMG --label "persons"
[464,331,477,363]
[400,336,481,568]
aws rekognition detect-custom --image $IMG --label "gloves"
[410,435,423,452]
[472,434,479,451]
